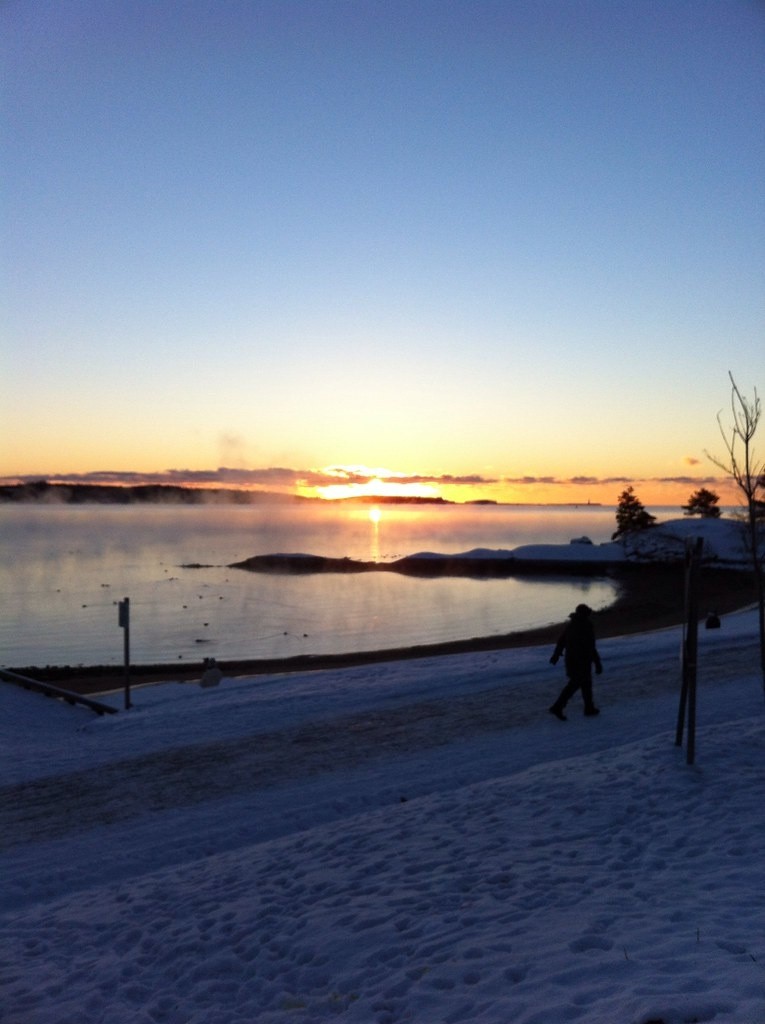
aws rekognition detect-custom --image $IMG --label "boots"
[550,701,567,721]
[584,699,600,716]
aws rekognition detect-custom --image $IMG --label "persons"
[548,603,603,721]
[705,610,721,629]
[200,657,222,687]
